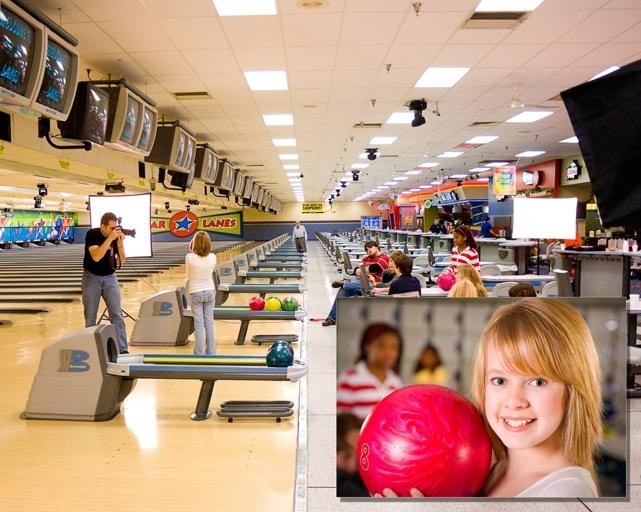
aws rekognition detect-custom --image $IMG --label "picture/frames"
[315,230,560,282]
[375,288,448,297]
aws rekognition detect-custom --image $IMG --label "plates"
[550,247,641,398]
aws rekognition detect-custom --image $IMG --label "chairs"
[322,318,335,326]
[332,281,343,288]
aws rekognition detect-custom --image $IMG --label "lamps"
[492,282,518,297]
[541,281,557,295]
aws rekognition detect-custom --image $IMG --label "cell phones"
[114,217,135,238]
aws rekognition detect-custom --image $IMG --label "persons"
[81,213,129,354]
[337,322,404,422]
[412,339,449,386]
[369,298,606,497]
[323,216,537,326]
[291,220,308,252]
[185,230,216,355]
[337,412,371,496]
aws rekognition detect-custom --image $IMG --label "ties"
[57,82,109,148]
[183,136,196,173]
[194,147,214,181]
[234,168,282,215]
[99,86,144,152]
[134,102,158,157]
[0,0,44,108]
[29,25,80,122]
[144,126,189,171]
[207,153,219,184]
[227,167,234,191]
[213,162,231,190]
